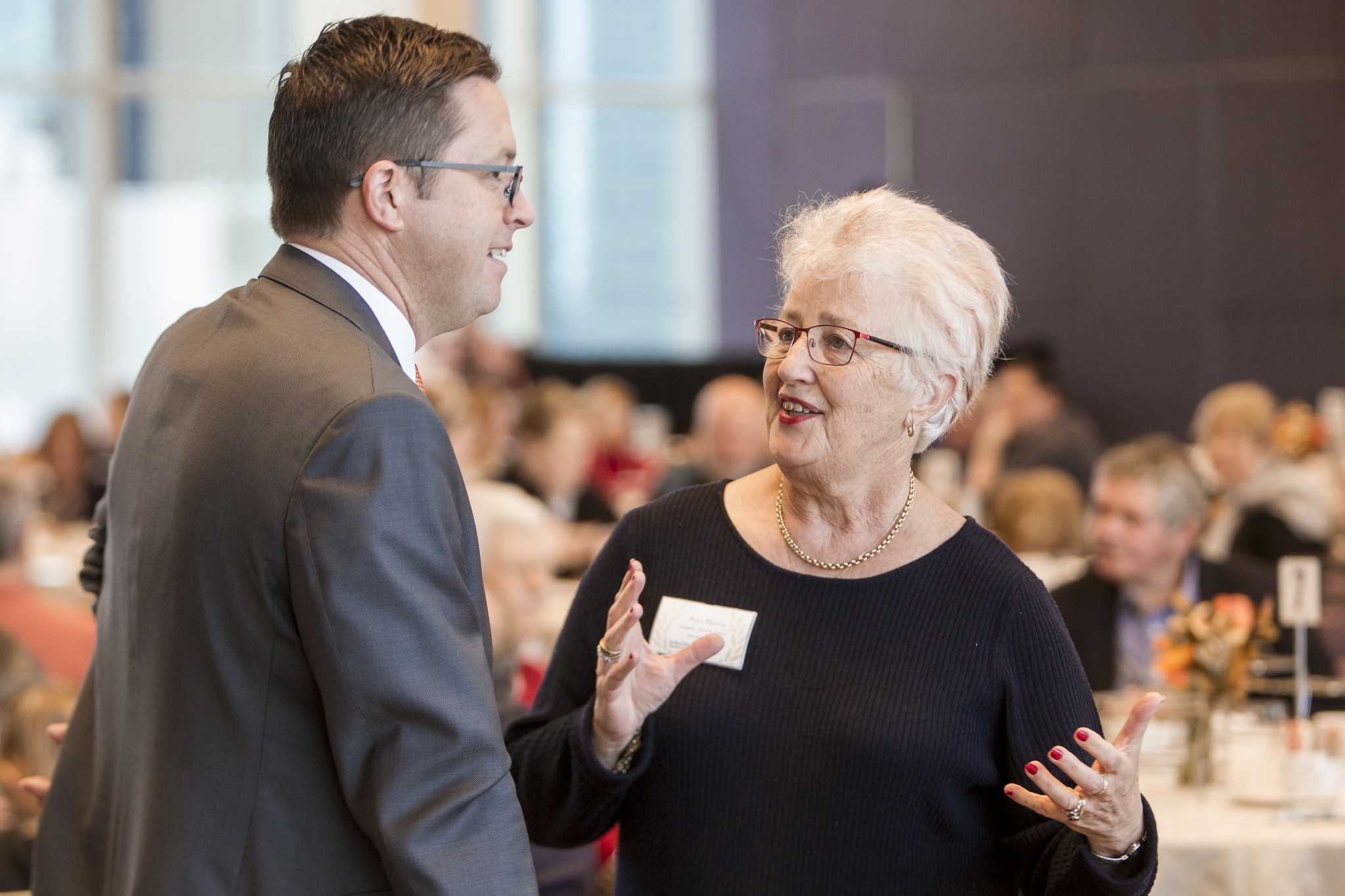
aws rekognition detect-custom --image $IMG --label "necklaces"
[775,466,915,570]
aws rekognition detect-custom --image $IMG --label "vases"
[1180,688,1213,786]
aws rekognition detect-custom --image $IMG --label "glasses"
[754,317,934,366]
[349,159,523,208]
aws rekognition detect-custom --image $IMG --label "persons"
[1,325,1345,896]
[33,13,538,896]
[504,185,1162,895]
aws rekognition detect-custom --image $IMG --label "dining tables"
[1100,708,1344,894]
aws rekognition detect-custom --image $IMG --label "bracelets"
[613,727,642,775]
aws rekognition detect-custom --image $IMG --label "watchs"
[1090,831,1149,864]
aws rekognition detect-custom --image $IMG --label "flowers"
[1148,594,1259,775]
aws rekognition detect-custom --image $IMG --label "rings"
[1087,771,1108,796]
[599,638,622,655]
[597,645,622,662]
[1062,790,1086,821]
